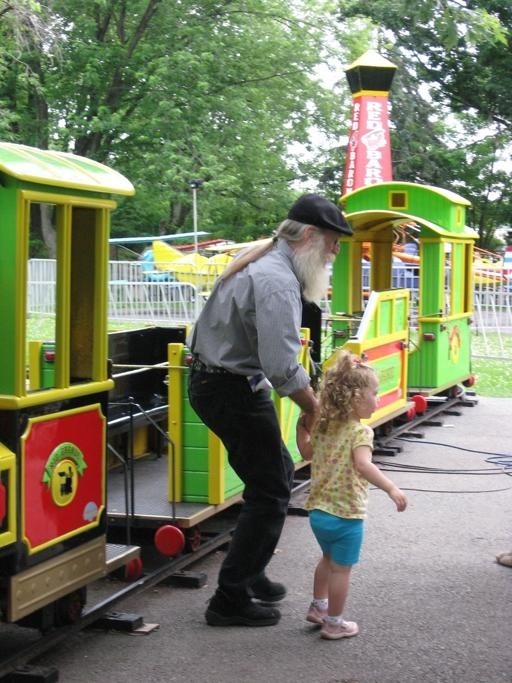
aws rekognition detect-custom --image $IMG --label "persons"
[186,192,352,627]
[296,352,407,639]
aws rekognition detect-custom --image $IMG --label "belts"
[192,363,235,375]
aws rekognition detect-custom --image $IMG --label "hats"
[287,193,355,237]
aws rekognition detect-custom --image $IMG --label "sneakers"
[243,569,287,602]
[205,589,281,627]
[319,617,359,641]
[306,602,329,624]
